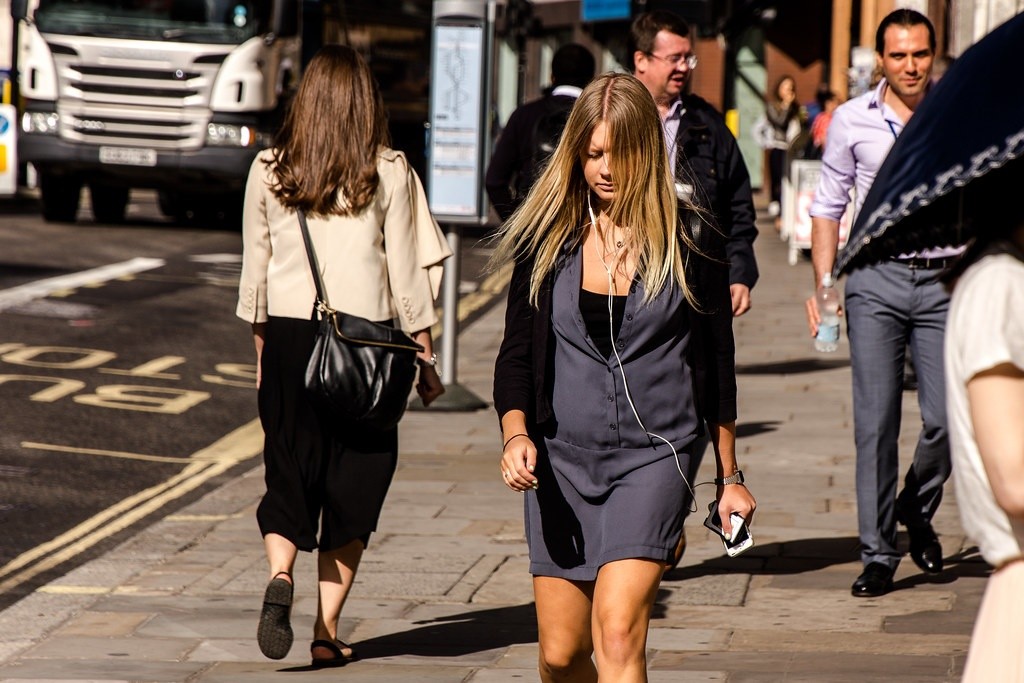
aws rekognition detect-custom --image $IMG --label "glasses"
[645,50,700,70]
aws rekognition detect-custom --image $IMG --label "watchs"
[714,469,745,486]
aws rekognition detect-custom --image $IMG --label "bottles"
[813,270,841,353]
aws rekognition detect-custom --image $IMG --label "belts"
[891,256,963,271]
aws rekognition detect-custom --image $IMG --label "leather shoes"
[896,498,943,576]
[850,561,896,597]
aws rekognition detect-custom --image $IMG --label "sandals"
[311,638,360,671]
[257,573,293,661]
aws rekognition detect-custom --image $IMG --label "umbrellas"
[830,9,1024,278]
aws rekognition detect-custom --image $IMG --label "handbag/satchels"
[304,309,424,430]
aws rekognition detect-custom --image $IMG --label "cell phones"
[709,500,754,558]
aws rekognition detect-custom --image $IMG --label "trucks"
[15,0,302,231]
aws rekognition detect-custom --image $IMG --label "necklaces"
[601,220,641,249]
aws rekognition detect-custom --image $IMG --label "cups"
[673,182,694,203]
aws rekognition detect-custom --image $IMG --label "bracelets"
[502,433,530,452]
[416,352,437,366]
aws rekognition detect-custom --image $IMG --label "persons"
[235,45,454,670]
[939,137,1024,683]
[485,18,760,441]
[762,76,842,231]
[487,71,758,683]
[804,8,957,598]
